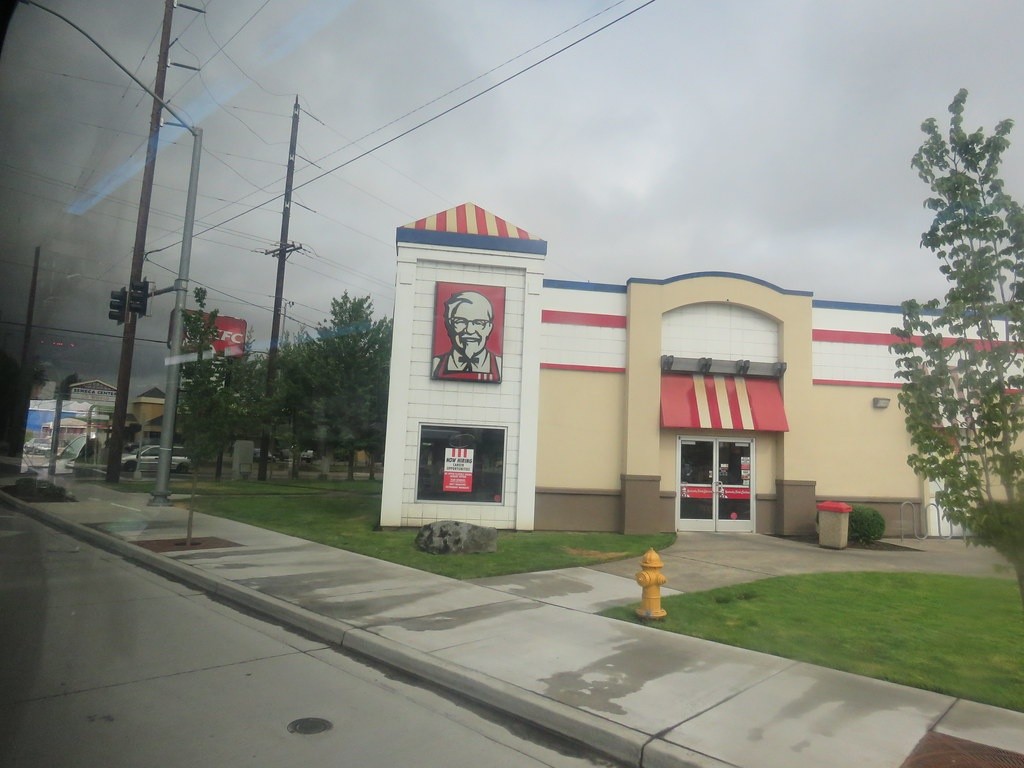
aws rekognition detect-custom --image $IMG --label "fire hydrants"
[635,547,668,620]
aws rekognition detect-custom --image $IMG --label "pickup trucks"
[278,444,315,463]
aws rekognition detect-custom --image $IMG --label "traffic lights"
[128,276,148,318]
[109,287,127,325]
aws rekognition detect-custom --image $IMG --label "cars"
[253,448,276,463]
[120,444,194,474]
[23,438,52,455]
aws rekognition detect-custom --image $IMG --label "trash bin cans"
[816,501,853,550]
